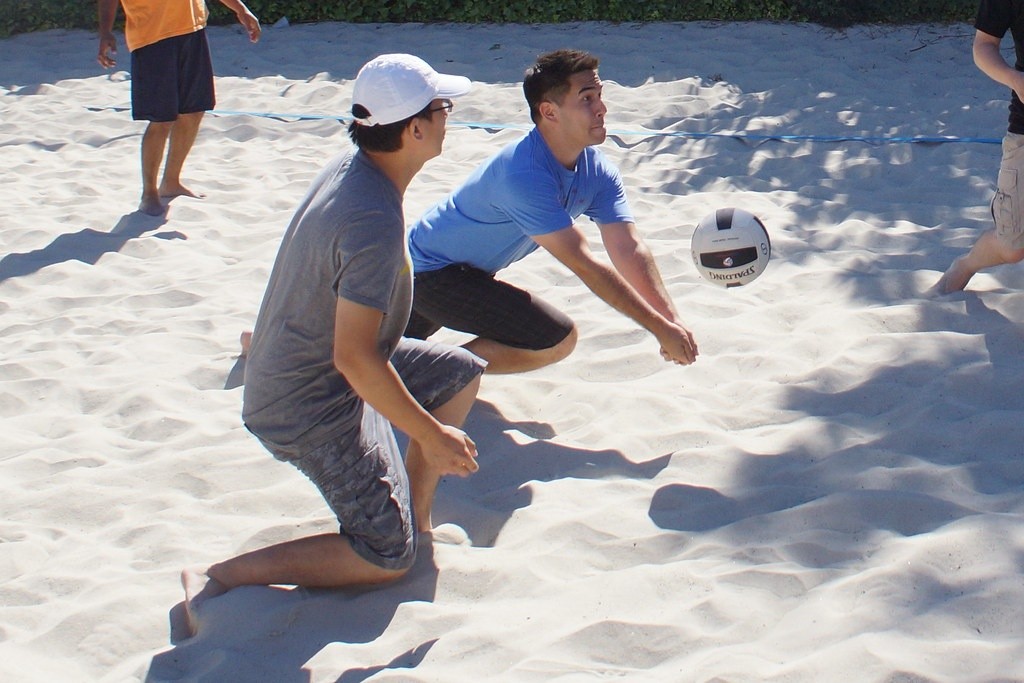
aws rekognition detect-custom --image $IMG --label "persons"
[240,51,699,375]
[182,54,489,634]
[924,0,1024,296]
[98,0,261,215]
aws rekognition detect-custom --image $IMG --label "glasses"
[429,98,454,112]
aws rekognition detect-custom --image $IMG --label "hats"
[350,54,471,125]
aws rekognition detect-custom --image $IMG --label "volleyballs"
[690,207,773,289]
